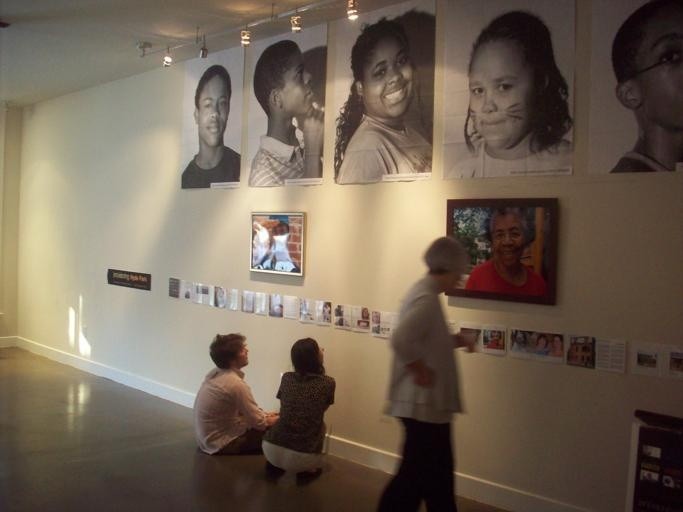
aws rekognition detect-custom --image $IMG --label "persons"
[249,40,324,187]
[334,19,431,183]
[251,220,270,267]
[264,222,297,274]
[481,332,561,356]
[261,337,336,485]
[609,1,682,172]
[444,10,571,174]
[193,332,281,457]
[181,63,240,187]
[378,237,471,512]
[466,207,544,299]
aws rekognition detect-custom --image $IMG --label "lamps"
[240,24,252,45]
[291,6,302,31]
[199,34,208,57]
[162,46,172,67]
[346,0,360,20]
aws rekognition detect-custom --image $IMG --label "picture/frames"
[249,213,305,277]
[444,199,557,306]
